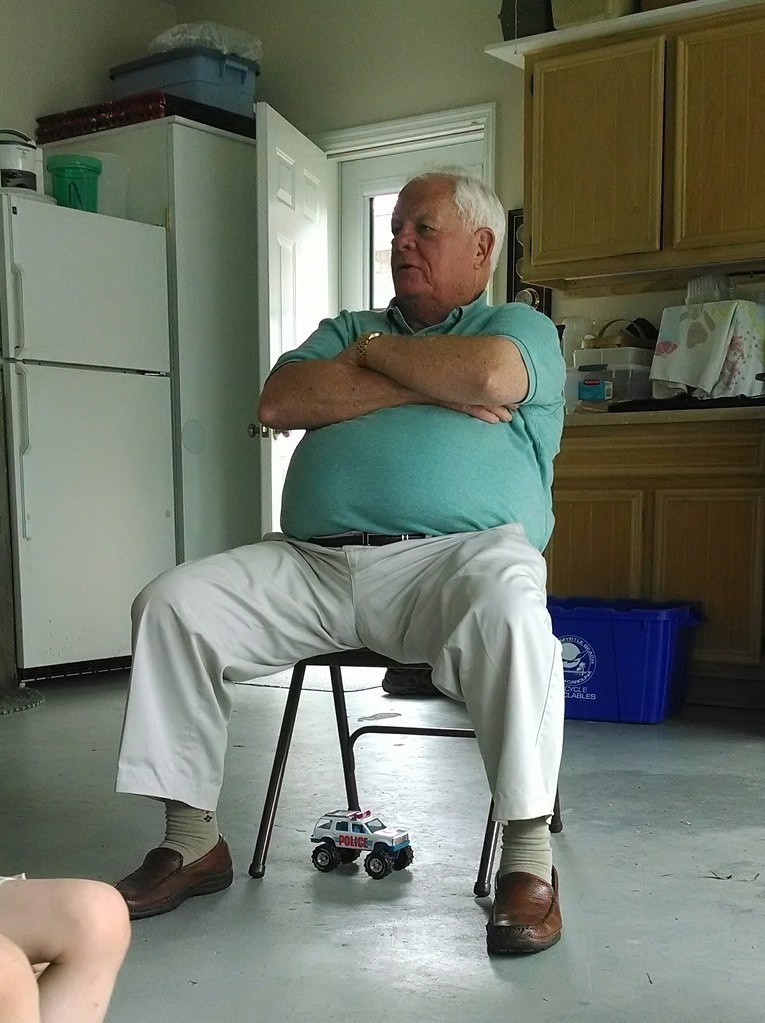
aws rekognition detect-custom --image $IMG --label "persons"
[0,873,132,1023]
[111,168,568,956]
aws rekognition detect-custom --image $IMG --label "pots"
[0,127,43,191]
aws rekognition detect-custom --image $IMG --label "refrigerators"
[1,192,177,689]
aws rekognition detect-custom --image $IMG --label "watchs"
[357,331,383,365]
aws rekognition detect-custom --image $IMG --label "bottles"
[576,363,615,413]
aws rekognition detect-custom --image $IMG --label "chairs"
[249,648,564,895]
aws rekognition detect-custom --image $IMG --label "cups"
[47,154,102,214]
[89,153,131,218]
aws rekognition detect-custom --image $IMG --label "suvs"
[310,811,414,880]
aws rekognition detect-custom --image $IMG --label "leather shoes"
[487,866,561,955]
[109,834,236,917]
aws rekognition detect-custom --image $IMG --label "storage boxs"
[47,154,102,213]
[562,345,655,417]
[109,47,261,119]
[547,594,699,724]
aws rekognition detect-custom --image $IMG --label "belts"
[307,530,429,546]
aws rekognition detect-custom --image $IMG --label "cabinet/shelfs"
[543,419,765,709]
[37,115,258,566]
[522,2,765,301]
